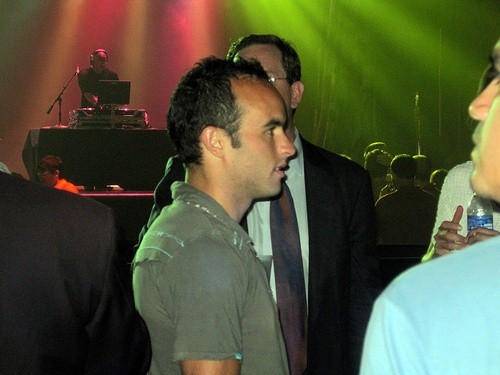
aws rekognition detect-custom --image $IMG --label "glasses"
[266,73,291,85]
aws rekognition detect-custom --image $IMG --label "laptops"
[97,80,131,104]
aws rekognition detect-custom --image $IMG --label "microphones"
[76,64,80,74]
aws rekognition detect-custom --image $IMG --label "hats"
[390,154,416,174]
[365,149,393,170]
[365,142,386,154]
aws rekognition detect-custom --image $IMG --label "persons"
[420,64,500,263]
[78,49,118,108]
[336,139,448,205]
[0,156,152,375]
[359,39,500,375]
[132,55,296,375]
[134,34,379,375]
[37,155,79,195]
[374,154,438,289]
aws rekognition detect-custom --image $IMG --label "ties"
[270,179,308,375]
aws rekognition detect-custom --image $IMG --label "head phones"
[90,50,107,65]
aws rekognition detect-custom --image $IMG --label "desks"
[21,128,178,191]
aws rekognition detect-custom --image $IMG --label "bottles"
[466,193,493,230]
